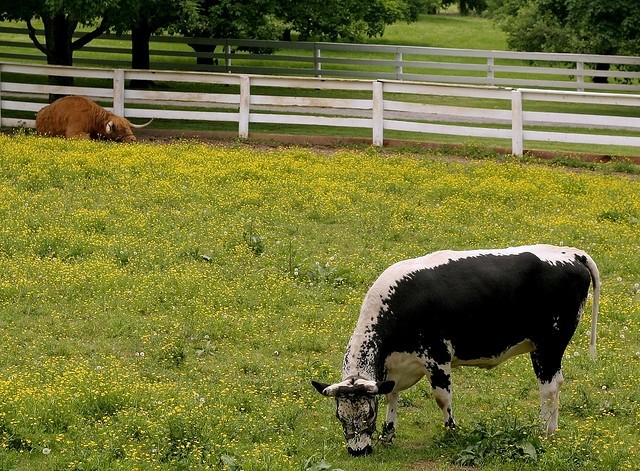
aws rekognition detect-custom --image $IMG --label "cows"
[312,244,600,457]
[36,96,154,144]
